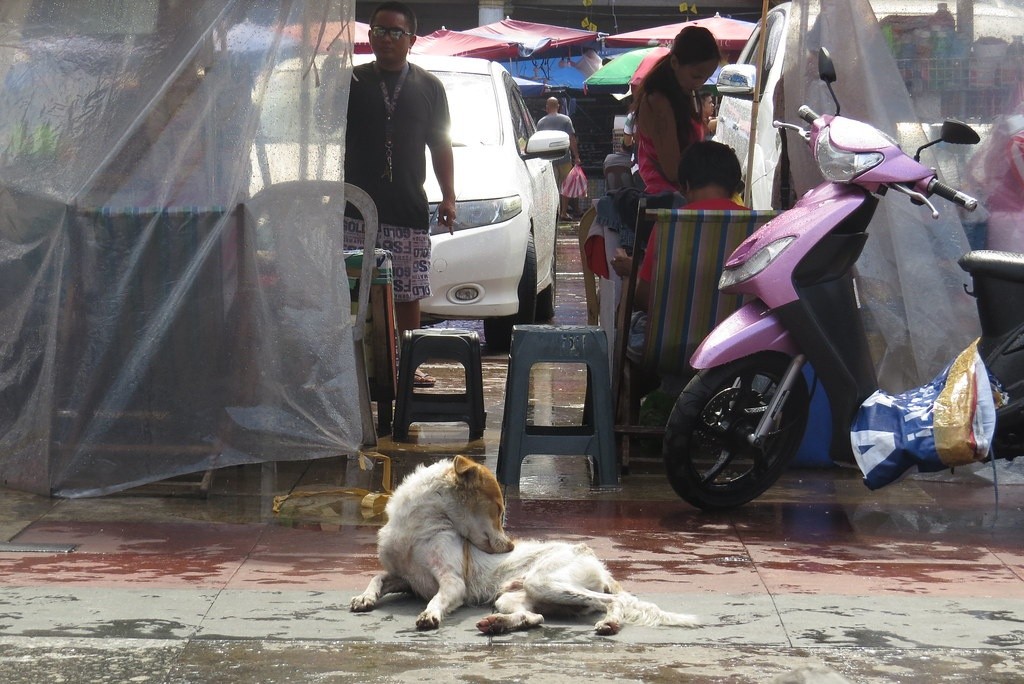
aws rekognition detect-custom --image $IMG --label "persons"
[346,0,457,386]
[536,96,582,220]
[626,26,754,370]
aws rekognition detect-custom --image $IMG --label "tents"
[187,12,759,101]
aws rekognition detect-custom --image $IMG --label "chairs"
[248,180,377,448]
[608,196,790,472]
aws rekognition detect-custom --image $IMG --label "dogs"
[351,454,700,636]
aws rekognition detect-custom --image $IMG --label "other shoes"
[560,214,574,224]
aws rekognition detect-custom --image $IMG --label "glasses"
[370,25,412,41]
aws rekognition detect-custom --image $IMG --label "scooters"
[662,113,1019,510]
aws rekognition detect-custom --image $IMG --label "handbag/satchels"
[559,164,588,199]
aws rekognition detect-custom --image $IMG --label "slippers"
[413,373,434,387]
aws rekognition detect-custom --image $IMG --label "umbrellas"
[584,45,670,93]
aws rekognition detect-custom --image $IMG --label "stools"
[497,325,623,491]
[395,326,487,442]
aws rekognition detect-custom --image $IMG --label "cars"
[241,51,570,347]
[714,2,1024,267]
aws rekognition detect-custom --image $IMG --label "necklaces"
[374,61,410,182]
